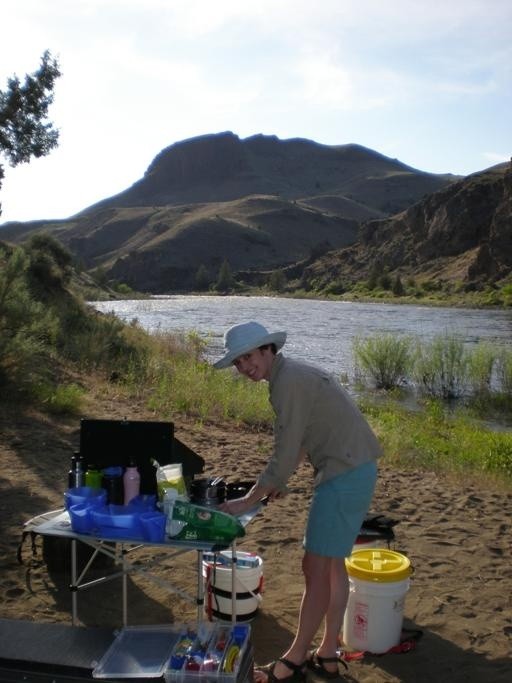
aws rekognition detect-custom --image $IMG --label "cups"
[69,503,96,533]
[64,487,108,513]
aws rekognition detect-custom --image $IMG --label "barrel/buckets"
[201,550,266,626]
[344,546,418,657]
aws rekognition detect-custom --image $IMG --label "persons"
[213,321,384,681]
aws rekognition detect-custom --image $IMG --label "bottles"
[84,463,102,492]
[124,465,140,508]
[67,451,83,491]
[105,466,123,506]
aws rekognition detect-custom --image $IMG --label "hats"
[213,321,286,370]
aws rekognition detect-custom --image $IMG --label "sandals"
[311,649,340,678]
[256,658,308,682]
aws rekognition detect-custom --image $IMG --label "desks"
[24,504,263,629]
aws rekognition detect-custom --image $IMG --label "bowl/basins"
[221,481,271,508]
[92,503,144,540]
[190,478,225,508]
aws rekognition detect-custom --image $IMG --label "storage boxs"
[44,536,115,574]
[91,621,253,683]
